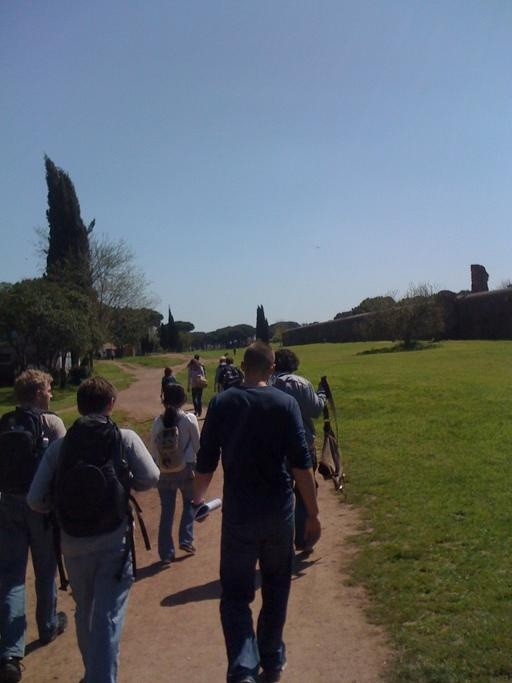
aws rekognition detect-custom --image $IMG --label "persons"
[219,357,245,389]
[0,369,67,683]
[148,382,201,565]
[187,359,208,417]
[214,357,226,392]
[160,368,177,397]
[26,376,159,683]
[194,354,200,361]
[270,349,326,551]
[191,342,321,683]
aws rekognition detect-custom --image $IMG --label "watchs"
[191,499,205,508]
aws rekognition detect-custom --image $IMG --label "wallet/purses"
[194,498,222,520]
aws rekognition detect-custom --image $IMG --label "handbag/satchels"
[196,366,208,388]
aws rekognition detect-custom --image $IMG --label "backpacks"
[222,366,244,387]
[0,406,58,526]
[48,414,134,536]
[154,411,191,473]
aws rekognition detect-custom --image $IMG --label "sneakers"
[41,611,67,644]
[1,657,22,681]
[179,543,196,554]
[264,657,287,681]
